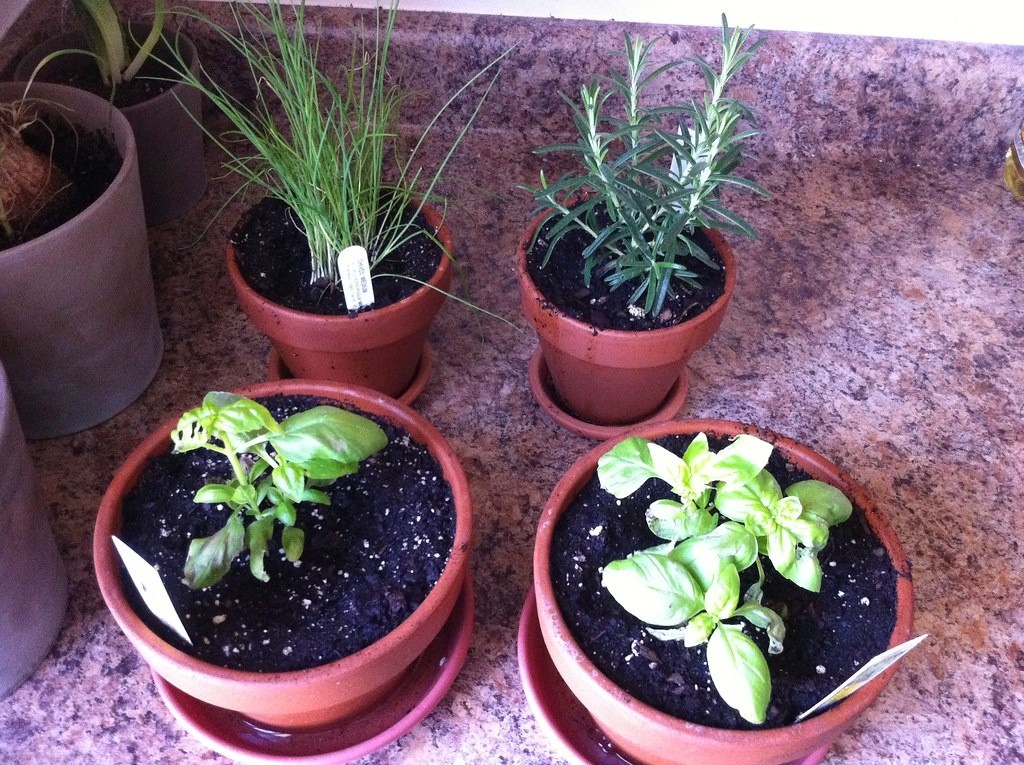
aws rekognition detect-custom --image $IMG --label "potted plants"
[508,11,779,448]
[0,79,166,442]
[8,0,210,231]
[506,415,930,765]
[92,379,476,765]
[126,0,459,411]
